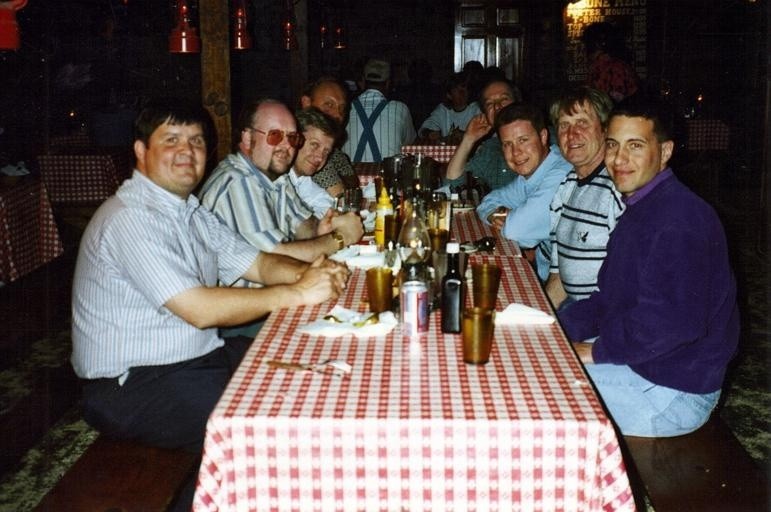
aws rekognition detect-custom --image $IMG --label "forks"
[266,359,352,372]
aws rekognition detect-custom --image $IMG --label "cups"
[462,307,494,365]
[471,264,500,310]
[366,268,392,312]
[375,177,391,203]
[427,228,447,251]
[385,215,400,249]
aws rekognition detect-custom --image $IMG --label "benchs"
[620,412,770,511]
[27,434,188,511]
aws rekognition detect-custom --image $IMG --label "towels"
[364,208,393,231]
[492,302,556,329]
[296,304,399,342]
[331,241,410,277]
[358,181,377,200]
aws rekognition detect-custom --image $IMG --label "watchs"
[330,229,344,250]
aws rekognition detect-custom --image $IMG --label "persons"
[557,96,740,436]
[68,95,349,450]
[300,75,360,198]
[442,77,522,194]
[474,102,574,279]
[289,106,338,219]
[198,97,364,341]
[343,58,417,164]
[418,78,480,142]
[544,86,625,343]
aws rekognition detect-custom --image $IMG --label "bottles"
[442,242,466,333]
[376,186,394,251]
[395,184,404,220]
[399,279,430,333]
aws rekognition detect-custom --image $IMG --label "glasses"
[248,125,306,150]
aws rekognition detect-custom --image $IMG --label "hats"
[361,59,390,82]
